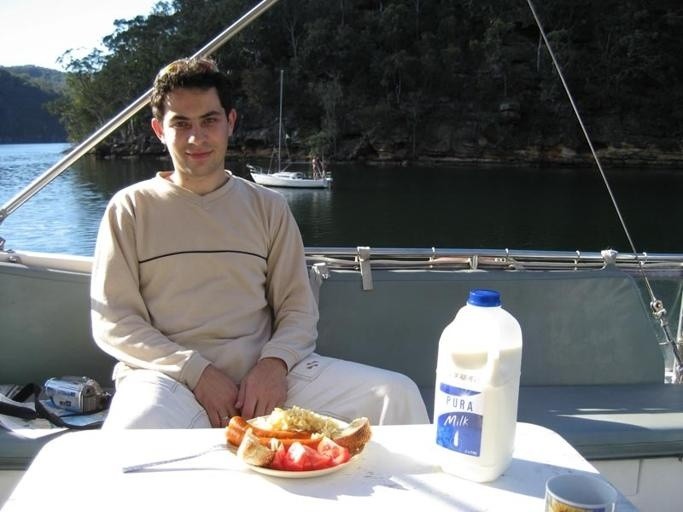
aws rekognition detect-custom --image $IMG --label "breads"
[226,416,373,452]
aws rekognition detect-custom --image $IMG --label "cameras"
[44,375,111,412]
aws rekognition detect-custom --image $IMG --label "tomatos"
[271,437,351,471]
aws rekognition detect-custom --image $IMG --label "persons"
[88,57,431,429]
[312,153,326,179]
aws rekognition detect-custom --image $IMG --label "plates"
[225,412,366,479]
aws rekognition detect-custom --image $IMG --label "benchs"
[0,263,683,466]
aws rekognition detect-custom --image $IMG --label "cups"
[545,473,615,512]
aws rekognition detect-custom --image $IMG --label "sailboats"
[245,66,335,190]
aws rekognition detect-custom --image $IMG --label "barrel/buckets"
[434,289,522,485]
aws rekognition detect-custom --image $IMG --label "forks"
[122,445,238,472]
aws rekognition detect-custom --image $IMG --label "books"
[0,393,109,441]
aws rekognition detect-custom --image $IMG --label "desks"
[2,424,638,512]
